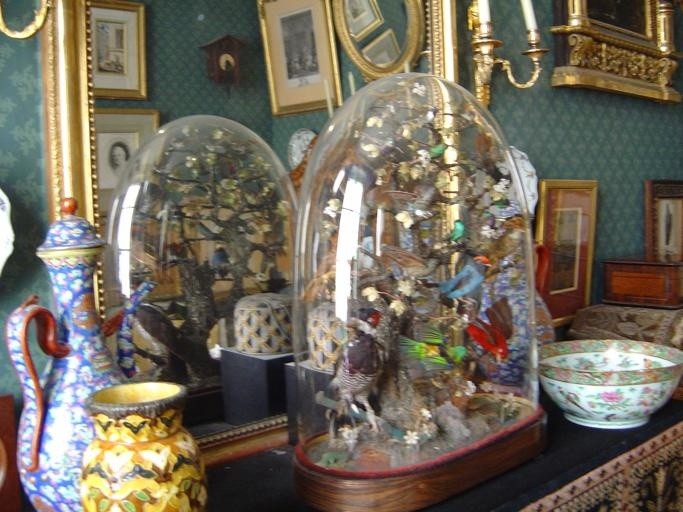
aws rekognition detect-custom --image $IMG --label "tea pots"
[4,196,158,512]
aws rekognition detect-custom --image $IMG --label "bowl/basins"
[536,339,683,429]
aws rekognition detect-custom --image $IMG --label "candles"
[520,0,540,30]
[477,1,491,24]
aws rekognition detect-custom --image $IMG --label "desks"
[182,307,682,511]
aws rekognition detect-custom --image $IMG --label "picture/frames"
[255,0,343,116]
[343,0,401,69]
[91,0,147,102]
[530,178,600,330]
[645,178,683,262]
[95,107,162,218]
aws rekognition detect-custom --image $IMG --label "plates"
[286,128,316,172]
[474,145,539,229]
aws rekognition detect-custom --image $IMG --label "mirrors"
[330,0,427,79]
